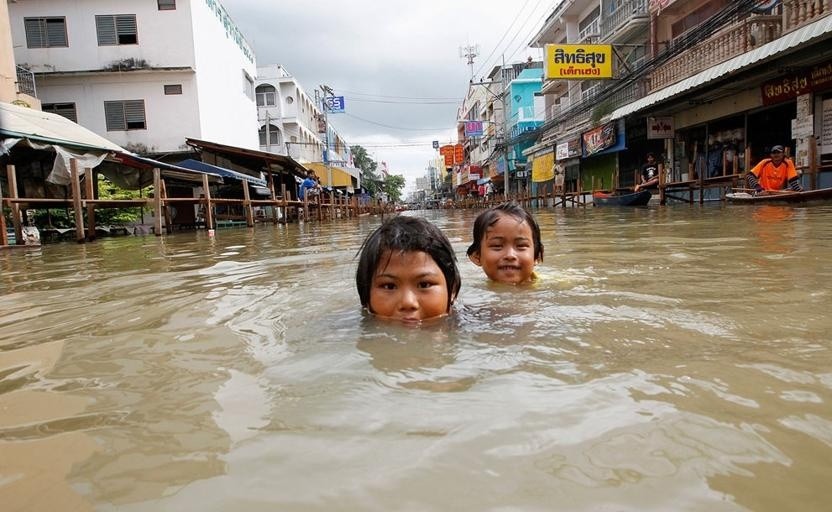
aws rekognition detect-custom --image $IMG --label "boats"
[723,187,832,205]
[591,190,652,206]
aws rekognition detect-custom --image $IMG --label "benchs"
[215,213,247,230]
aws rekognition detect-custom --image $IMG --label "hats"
[771,145,784,153]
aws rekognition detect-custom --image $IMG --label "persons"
[298,169,323,205]
[634,152,659,192]
[745,145,805,196]
[466,201,544,289]
[551,160,565,192]
[478,180,497,202]
[356,215,461,326]
